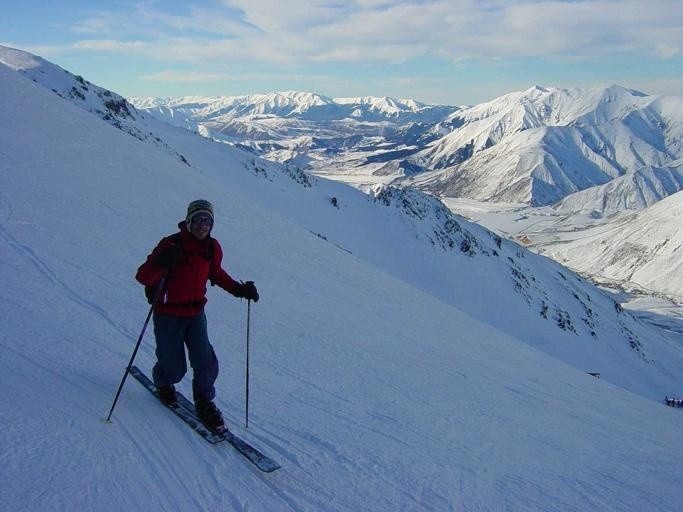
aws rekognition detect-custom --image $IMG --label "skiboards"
[127,365,281,473]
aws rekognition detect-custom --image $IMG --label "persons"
[135,198,259,435]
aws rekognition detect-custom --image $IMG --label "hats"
[185,200,214,222]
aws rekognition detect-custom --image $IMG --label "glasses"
[192,216,213,226]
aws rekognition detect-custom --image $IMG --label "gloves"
[235,281,259,302]
[145,286,159,304]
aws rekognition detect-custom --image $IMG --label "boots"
[152,367,177,404]
[193,388,224,429]
